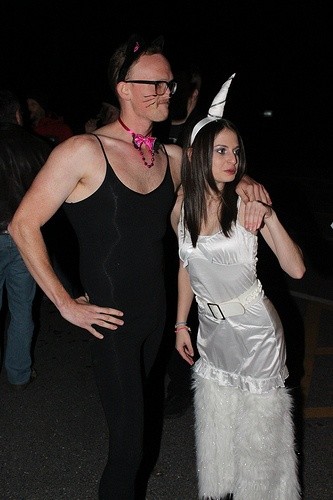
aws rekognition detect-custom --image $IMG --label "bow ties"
[117,116,157,151]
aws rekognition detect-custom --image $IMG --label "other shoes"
[11,368,38,392]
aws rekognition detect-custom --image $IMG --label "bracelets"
[173,321,193,333]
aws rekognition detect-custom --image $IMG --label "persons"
[0,92,45,388]
[7,39,274,500]
[14,95,120,141]
[169,113,309,500]
[156,63,201,146]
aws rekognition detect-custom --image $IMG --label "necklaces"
[117,113,158,169]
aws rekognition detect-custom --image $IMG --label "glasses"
[119,79,177,96]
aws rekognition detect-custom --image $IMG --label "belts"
[196,280,262,320]
